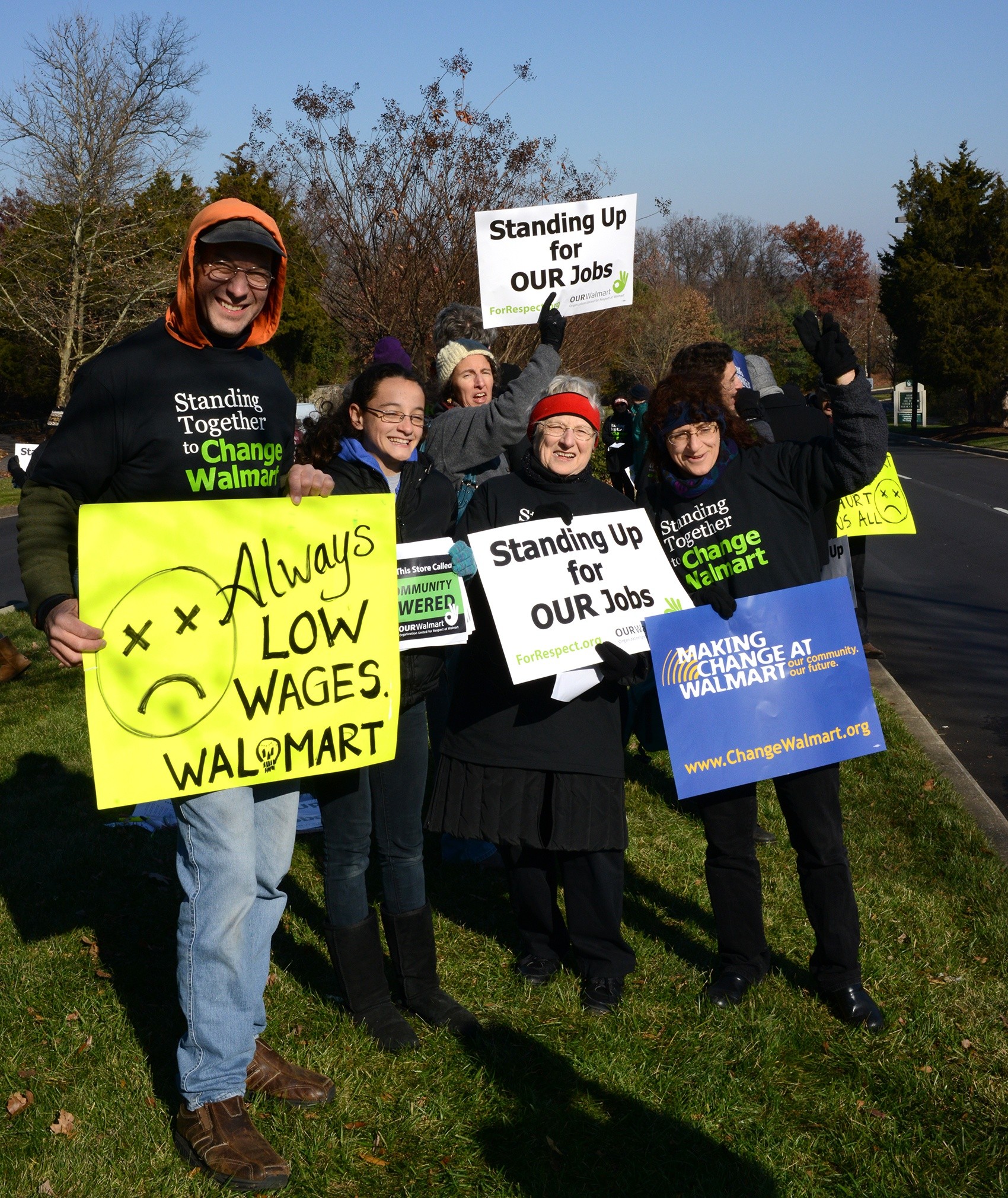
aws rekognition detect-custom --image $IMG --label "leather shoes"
[709,968,752,1009]
[822,982,886,1033]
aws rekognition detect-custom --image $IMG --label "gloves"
[449,540,478,582]
[530,501,573,526]
[704,585,738,620]
[595,641,639,679]
[735,387,762,421]
[538,292,568,353]
[793,309,858,380]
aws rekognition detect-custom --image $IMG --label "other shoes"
[246,1034,338,1110]
[515,954,559,984]
[176,1094,293,1190]
[753,825,774,842]
[580,976,624,1016]
[863,642,887,659]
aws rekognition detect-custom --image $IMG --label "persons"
[19,196,338,1194]
[296,292,892,1052]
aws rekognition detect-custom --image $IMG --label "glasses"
[664,423,720,445]
[202,257,277,288]
[538,422,597,441]
[615,402,626,408]
[357,405,432,427]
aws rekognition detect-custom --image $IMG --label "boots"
[327,907,424,1054]
[381,912,486,1038]
[0,635,33,683]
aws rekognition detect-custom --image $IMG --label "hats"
[631,385,650,401]
[198,218,288,260]
[744,355,777,391]
[373,337,413,372]
[432,338,497,393]
[732,351,753,390]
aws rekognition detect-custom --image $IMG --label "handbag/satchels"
[454,470,477,525]
